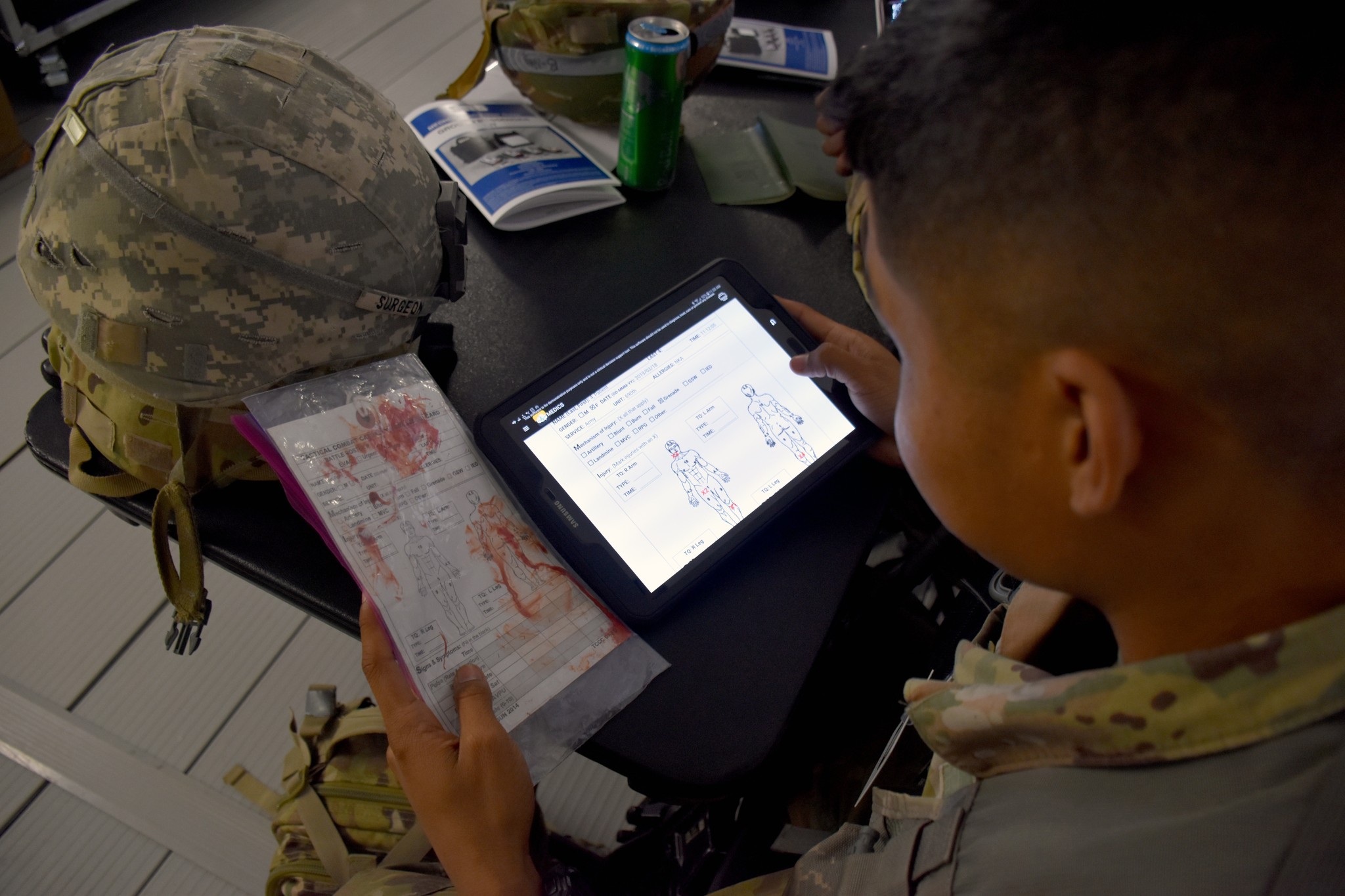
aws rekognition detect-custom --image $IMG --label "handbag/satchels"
[223,682,463,896]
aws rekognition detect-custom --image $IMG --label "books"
[685,116,850,208]
[397,96,627,233]
[713,14,840,84]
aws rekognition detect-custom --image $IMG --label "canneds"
[616,17,689,196]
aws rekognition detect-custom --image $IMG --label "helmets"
[37,325,459,657]
[17,24,471,410]
[435,0,735,130]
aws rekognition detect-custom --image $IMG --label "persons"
[362,0,1345,896]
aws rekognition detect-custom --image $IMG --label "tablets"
[472,258,885,632]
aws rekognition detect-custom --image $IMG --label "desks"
[22,0,900,800]
